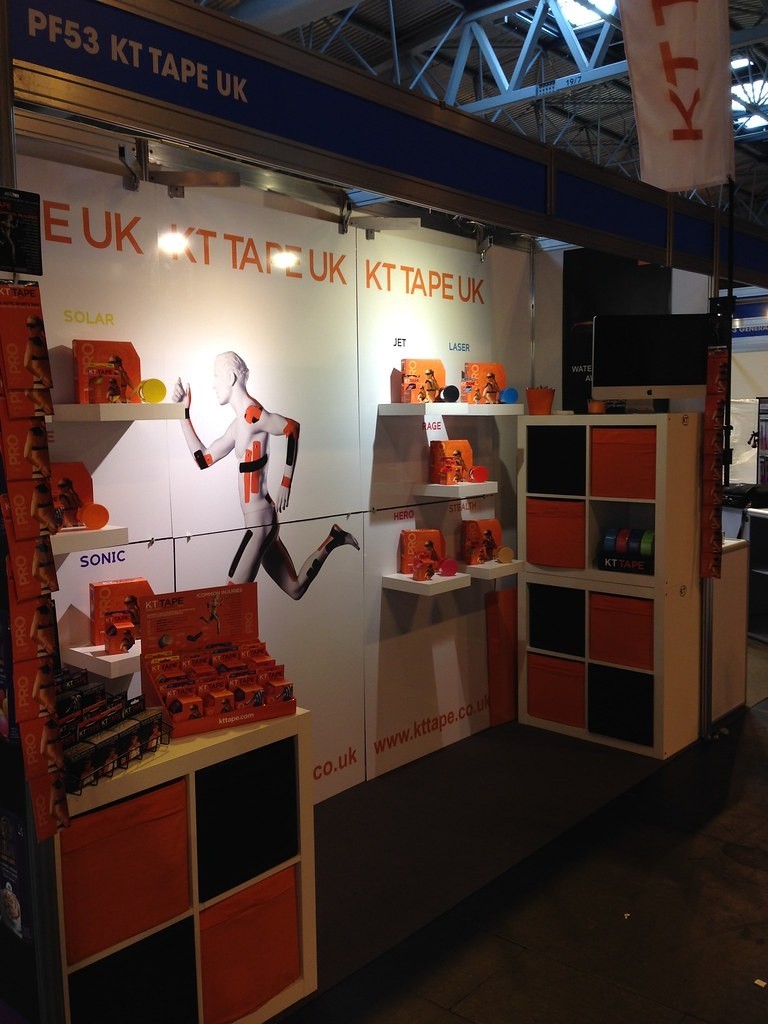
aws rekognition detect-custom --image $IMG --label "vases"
[526,385,556,416]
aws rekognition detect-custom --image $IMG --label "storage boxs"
[44,341,657,1024]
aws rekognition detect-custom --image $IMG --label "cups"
[526,388,555,415]
[585,382,606,413]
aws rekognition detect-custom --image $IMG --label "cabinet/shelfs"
[46,404,186,672]
[54,706,318,1024]
[517,412,699,761]
[722,506,768,645]
[712,537,750,728]
[378,403,518,597]
